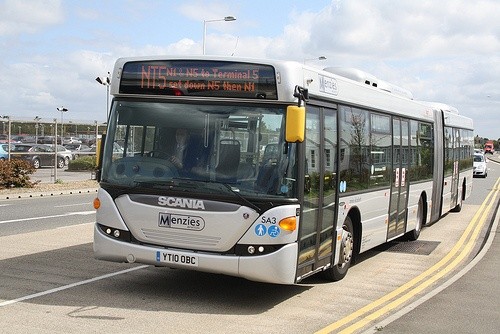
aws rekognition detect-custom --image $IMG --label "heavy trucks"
[484,140,494,155]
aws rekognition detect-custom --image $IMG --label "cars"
[0,143,11,162]
[10,144,66,169]
[0,133,128,148]
[473,153,488,179]
[474,149,484,155]
[71,145,124,161]
[64,142,89,161]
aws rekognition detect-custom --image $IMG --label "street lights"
[2,114,99,182]
[57,106,69,142]
[94,71,111,121]
[203,14,237,55]
[304,55,327,65]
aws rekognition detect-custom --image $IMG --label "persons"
[157,128,198,178]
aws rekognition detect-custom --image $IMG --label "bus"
[91,54,475,286]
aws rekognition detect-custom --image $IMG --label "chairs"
[213,139,240,184]
[255,144,284,194]
